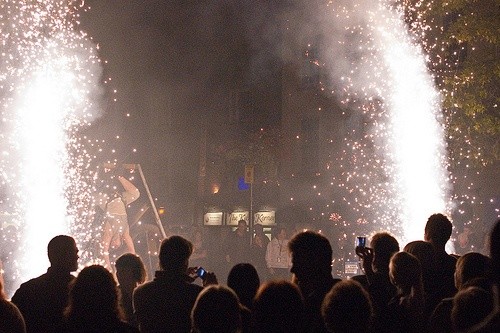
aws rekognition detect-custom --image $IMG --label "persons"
[91,163,140,273]
[191,213,500,333]
[1,233,218,332]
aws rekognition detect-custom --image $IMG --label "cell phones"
[195,267,207,279]
[356,236,365,253]
